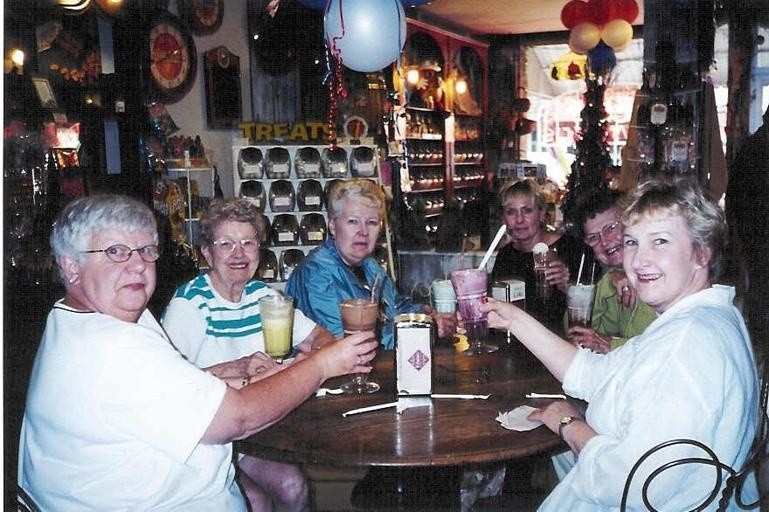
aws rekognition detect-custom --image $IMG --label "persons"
[161,199,335,511]
[487,182,598,353]
[285,178,513,510]
[568,187,658,354]
[18,196,376,510]
[476,180,762,510]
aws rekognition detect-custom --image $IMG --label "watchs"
[557,415,586,438]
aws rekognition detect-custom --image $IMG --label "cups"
[533,246,559,309]
[432,278,462,345]
[447,268,499,357]
[566,284,598,329]
[340,298,379,396]
[259,294,295,357]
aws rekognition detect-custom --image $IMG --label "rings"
[254,367,262,374]
[355,356,363,365]
[623,285,630,291]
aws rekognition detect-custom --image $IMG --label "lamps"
[456,69,468,93]
[404,49,420,85]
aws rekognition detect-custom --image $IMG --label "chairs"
[620,413,769,510]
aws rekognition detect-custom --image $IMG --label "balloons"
[323,0,406,72]
[559,0,640,55]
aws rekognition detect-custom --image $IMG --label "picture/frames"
[33,79,57,109]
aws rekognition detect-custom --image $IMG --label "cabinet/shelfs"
[392,18,490,242]
[158,165,215,255]
[232,146,397,303]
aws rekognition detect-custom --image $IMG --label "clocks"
[180,0,224,36]
[144,16,198,104]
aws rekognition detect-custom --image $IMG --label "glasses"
[582,221,622,248]
[503,207,536,219]
[78,244,162,264]
[210,237,262,253]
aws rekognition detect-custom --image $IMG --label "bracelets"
[240,376,251,385]
